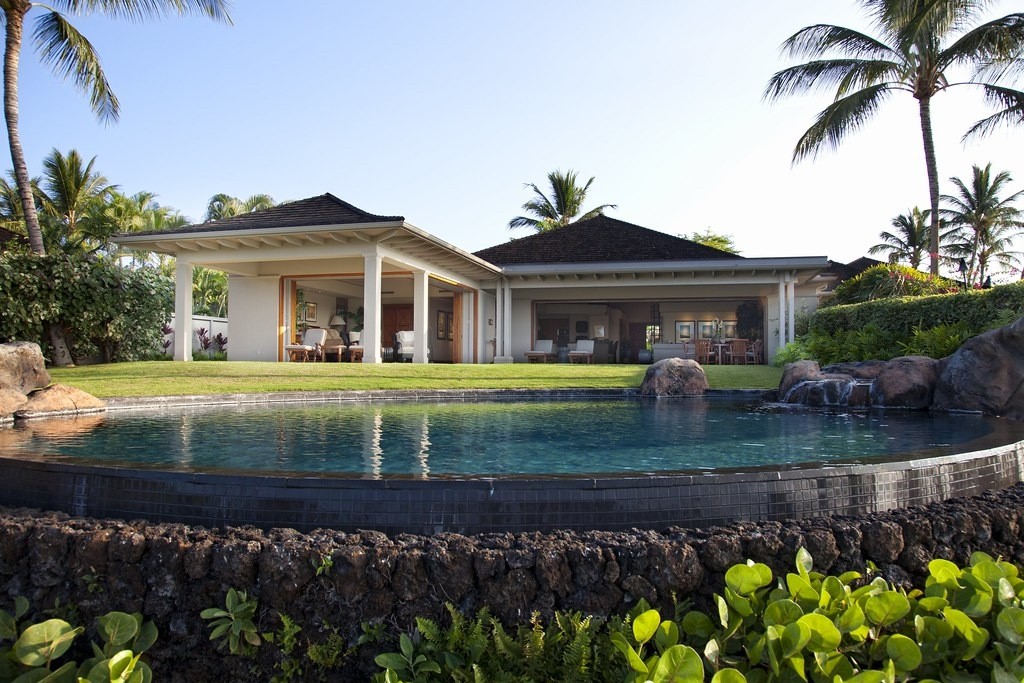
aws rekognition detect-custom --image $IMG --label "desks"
[321,346,347,363]
[710,344,731,365]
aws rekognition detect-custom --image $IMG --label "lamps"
[329,316,346,332]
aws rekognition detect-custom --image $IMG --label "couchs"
[393,330,431,363]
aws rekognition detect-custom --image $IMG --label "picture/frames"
[697,320,718,342]
[305,302,317,322]
[593,324,605,338]
[675,321,695,344]
[447,312,453,339]
[437,310,446,339]
[719,320,739,342]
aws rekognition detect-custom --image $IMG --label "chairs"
[568,339,595,364]
[285,328,327,363]
[691,337,763,365]
[524,340,554,365]
[348,329,365,363]
